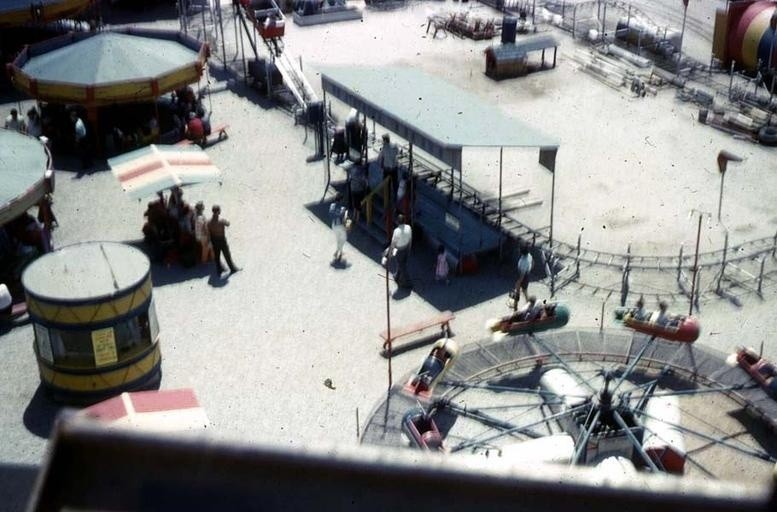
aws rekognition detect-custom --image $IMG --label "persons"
[327,108,417,228]
[34,190,59,243]
[631,297,671,326]
[4,82,211,168]
[495,245,562,323]
[328,191,353,264]
[382,214,412,292]
[434,244,450,287]
[141,185,240,276]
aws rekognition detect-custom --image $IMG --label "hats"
[527,294,536,302]
[195,200,203,209]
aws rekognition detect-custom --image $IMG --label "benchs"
[379,309,455,352]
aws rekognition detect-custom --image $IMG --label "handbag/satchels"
[201,246,209,263]
[345,219,352,231]
[508,289,520,300]
[381,256,389,266]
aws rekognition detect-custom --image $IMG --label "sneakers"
[333,252,342,260]
[217,265,243,272]
